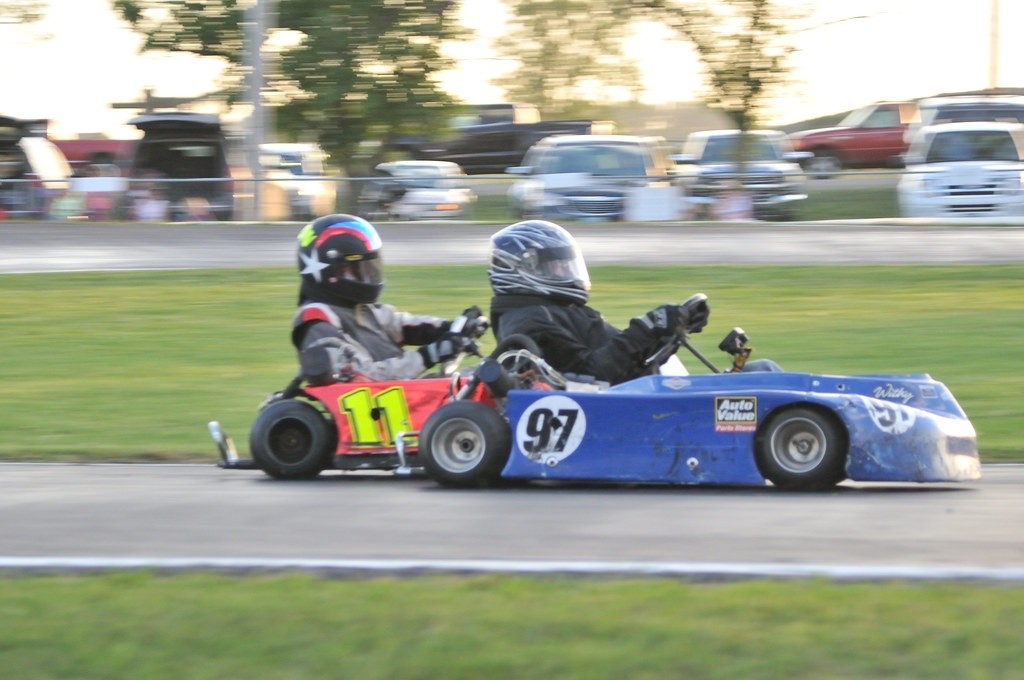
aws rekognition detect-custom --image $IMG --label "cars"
[887,118,1024,220]
[354,158,479,222]
[776,98,917,179]
[503,135,672,222]
[666,128,815,219]
[900,95,1024,145]
[0,109,341,224]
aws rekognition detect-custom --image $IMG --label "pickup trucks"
[370,112,593,176]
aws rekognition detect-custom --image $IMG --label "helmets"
[487,219,591,306]
[297,214,386,304]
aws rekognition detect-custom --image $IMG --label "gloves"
[441,304,488,339]
[629,304,692,344]
[417,330,474,369]
[688,304,709,335]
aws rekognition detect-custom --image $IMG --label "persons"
[0,149,211,220]
[290,214,485,383]
[487,220,709,386]
